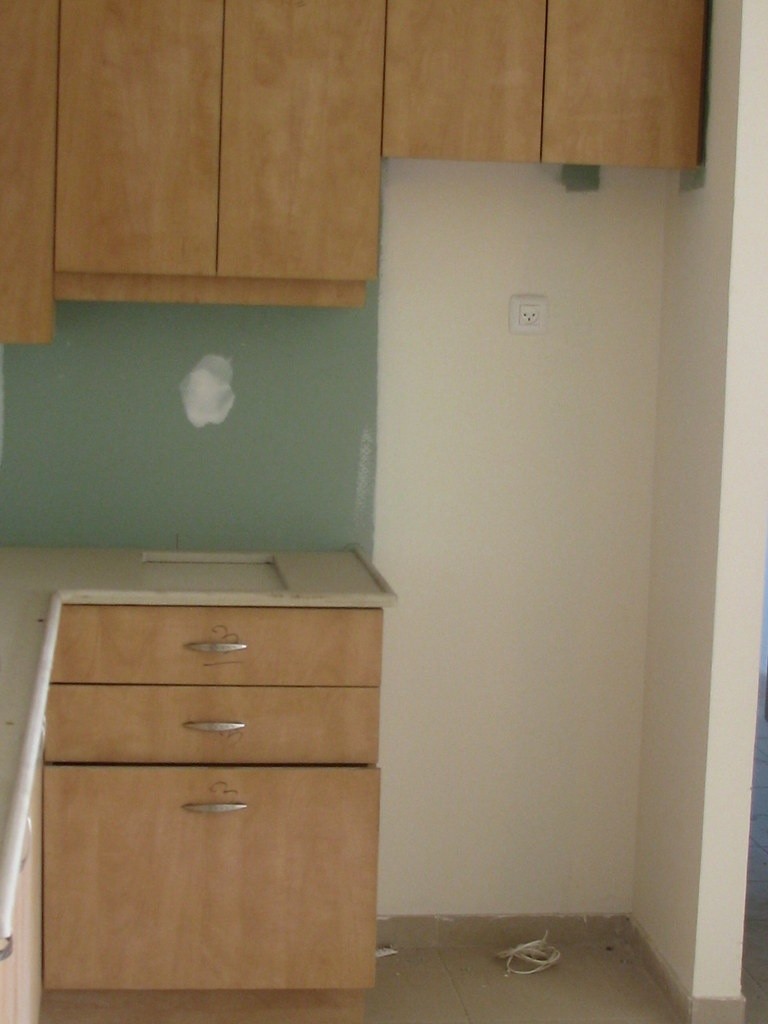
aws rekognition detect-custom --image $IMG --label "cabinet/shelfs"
[0,605,383,1024]
[0,0,706,342]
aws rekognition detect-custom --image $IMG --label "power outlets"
[509,294,548,336]
